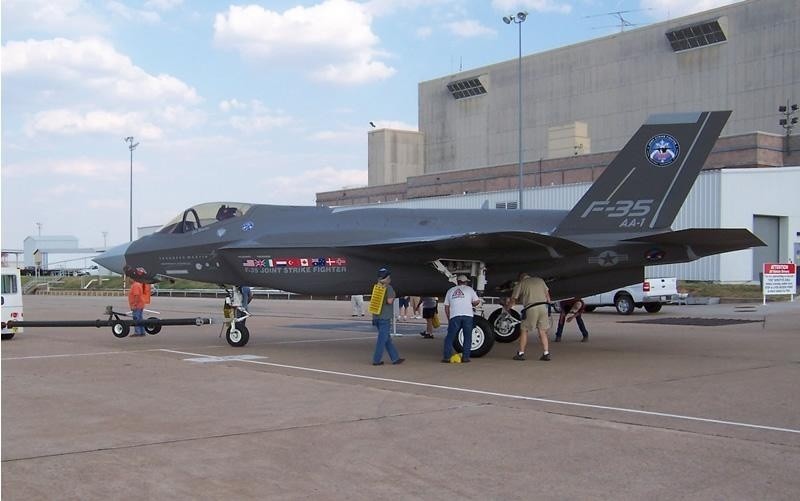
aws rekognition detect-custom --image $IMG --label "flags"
[243,258,347,267]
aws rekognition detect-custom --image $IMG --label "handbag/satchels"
[432,312,440,328]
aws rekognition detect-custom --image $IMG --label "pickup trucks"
[553,275,679,313]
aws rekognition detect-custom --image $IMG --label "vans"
[1,266,25,338]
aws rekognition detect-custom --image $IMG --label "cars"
[21,263,98,278]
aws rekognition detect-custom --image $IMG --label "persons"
[127,278,146,337]
[351,294,365,316]
[397,296,410,321]
[555,299,588,343]
[410,296,421,319]
[371,267,405,366]
[414,297,438,339]
[506,270,553,361]
[237,287,254,327]
[440,274,480,364]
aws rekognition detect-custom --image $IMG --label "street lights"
[779,97,799,165]
[121,135,140,242]
[102,231,108,249]
[37,221,42,237]
[504,11,529,215]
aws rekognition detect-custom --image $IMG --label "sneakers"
[373,361,384,365]
[130,334,145,337]
[513,352,526,360]
[539,352,551,361]
[393,358,405,364]
[580,336,588,342]
[442,359,450,363]
[461,358,471,362]
[555,336,561,341]
[397,315,422,321]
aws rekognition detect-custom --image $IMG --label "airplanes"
[93,108,769,358]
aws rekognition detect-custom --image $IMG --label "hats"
[570,301,582,314]
[457,275,471,282]
[378,268,392,279]
